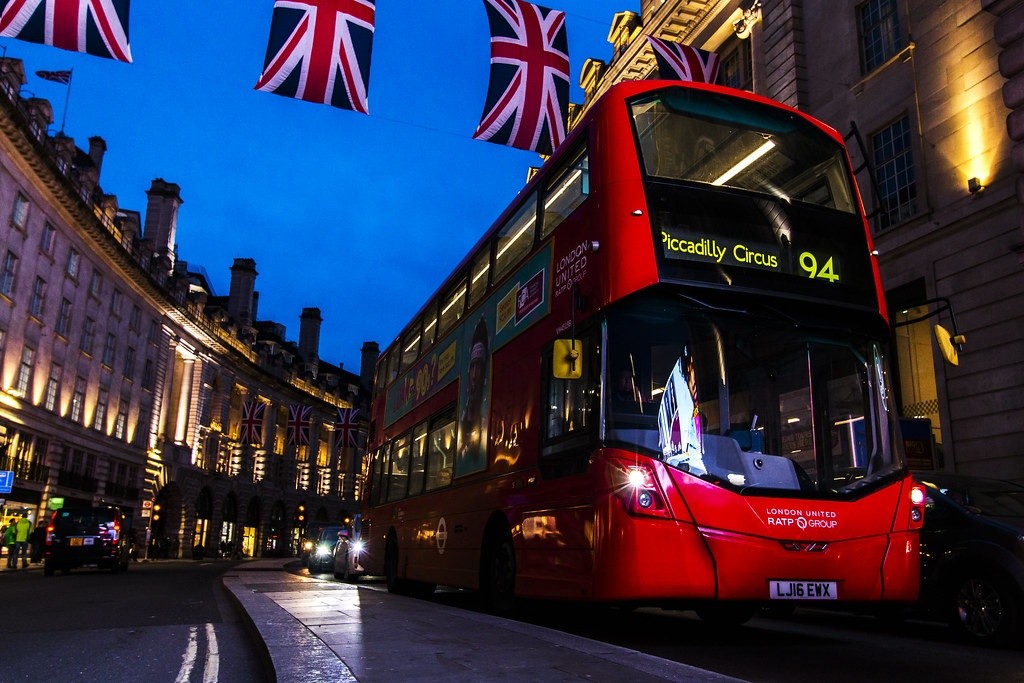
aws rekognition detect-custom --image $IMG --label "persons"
[456,319,487,461]
[220,541,234,559]
[0,513,47,569]
[131,534,180,562]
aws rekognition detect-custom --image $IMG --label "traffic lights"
[150,504,162,527]
[296,504,306,525]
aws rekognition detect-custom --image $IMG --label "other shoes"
[22,564,29,568]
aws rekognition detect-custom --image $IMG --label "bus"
[355,78,968,622]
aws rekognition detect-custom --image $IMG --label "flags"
[472,1,570,157]
[647,35,717,84]
[334,408,361,449]
[0,1,134,65]
[253,0,377,116]
[238,402,267,444]
[286,404,312,447]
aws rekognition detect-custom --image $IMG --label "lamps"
[965,176,987,207]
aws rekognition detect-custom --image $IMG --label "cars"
[837,467,1024,649]
[308,540,335,574]
[332,529,362,582]
[42,505,129,578]
